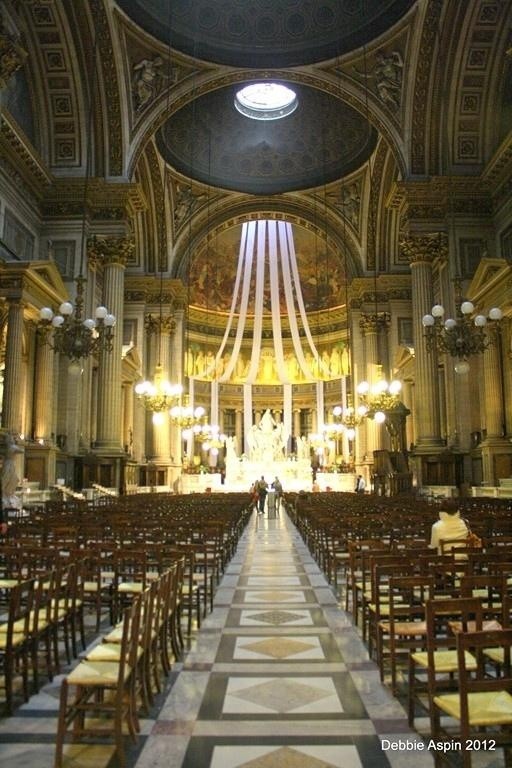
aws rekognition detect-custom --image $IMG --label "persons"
[428,500,469,589]
[312,462,366,494]
[132,57,178,111]
[185,462,226,484]
[352,51,404,110]
[249,476,283,515]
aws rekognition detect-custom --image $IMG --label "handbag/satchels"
[258,488,267,496]
[467,533,482,549]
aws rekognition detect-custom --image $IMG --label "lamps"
[38,0,512,462]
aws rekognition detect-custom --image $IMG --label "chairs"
[284,487,511,766]
[0,487,261,768]
